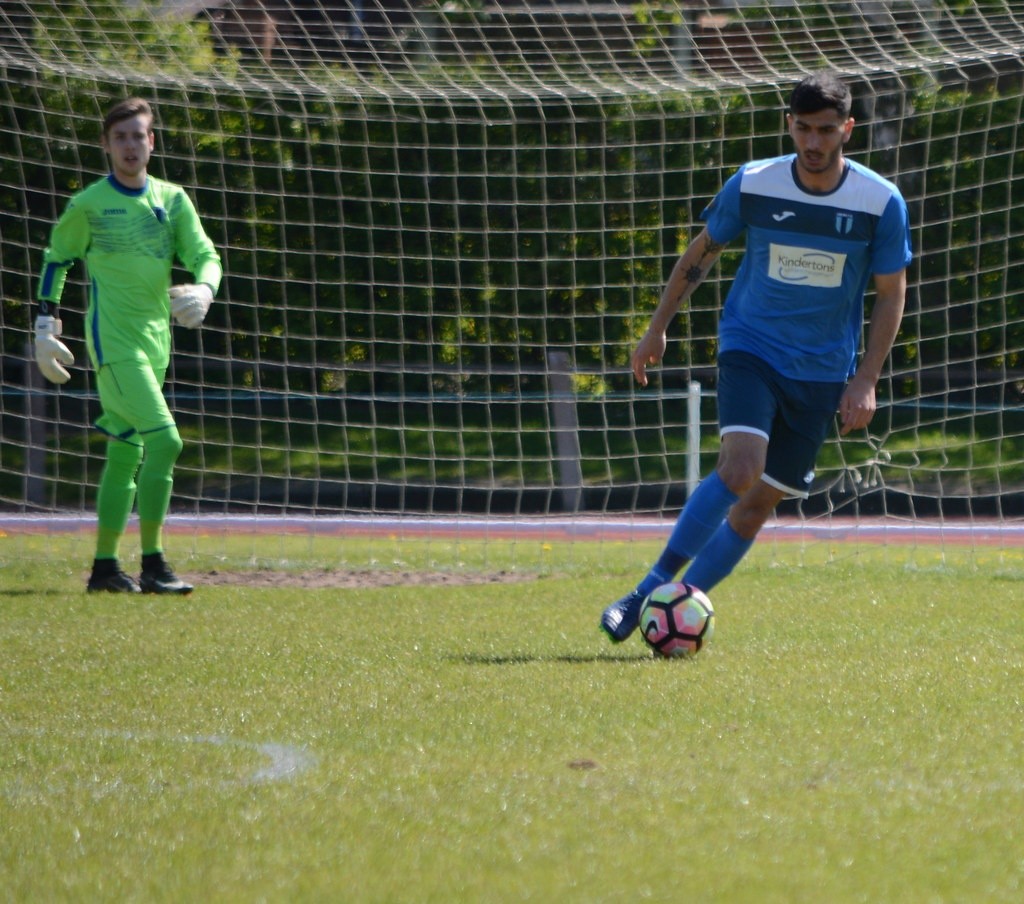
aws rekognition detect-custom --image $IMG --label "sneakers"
[599,589,646,644]
[139,571,195,594]
[87,571,142,594]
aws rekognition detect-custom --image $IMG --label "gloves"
[34,315,74,385]
[168,283,215,330]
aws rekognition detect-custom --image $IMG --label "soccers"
[638,581,716,660]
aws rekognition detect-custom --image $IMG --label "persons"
[602,70,913,654]
[35,99,223,595]
